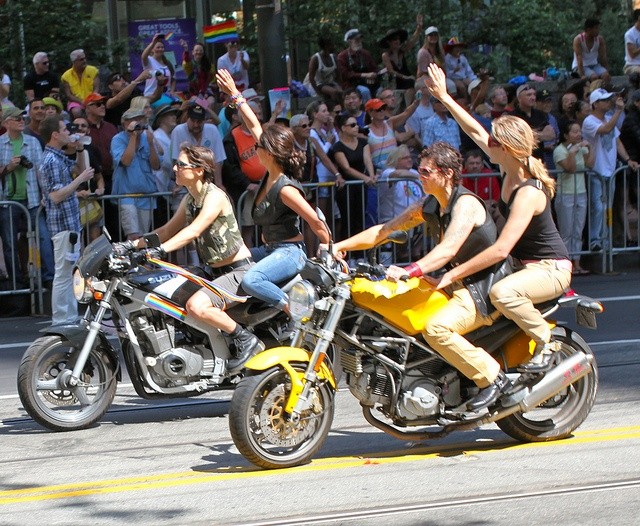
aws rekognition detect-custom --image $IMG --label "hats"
[241,88,265,104]
[347,32,363,40]
[538,89,553,103]
[448,36,466,48]
[83,91,107,109]
[152,104,178,130]
[377,28,408,49]
[424,26,439,36]
[120,109,145,123]
[589,88,616,105]
[364,98,387,113]
[188,106,205,121]
[42,97,63,114]
[468,80,482,95]
[0,106,27,123]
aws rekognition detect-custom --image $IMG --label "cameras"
[134,124,148,131]
[20,155,33,169]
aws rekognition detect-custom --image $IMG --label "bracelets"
[76,148,85,153]
[228,93,247,110]
[413,100,420,107]
[335,172,341,176]
[626,158,631,163]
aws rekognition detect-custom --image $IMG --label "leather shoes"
[466,370,511,412]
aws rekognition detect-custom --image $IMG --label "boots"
[227,325,266,374]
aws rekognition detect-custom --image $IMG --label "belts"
[211,257,254,274]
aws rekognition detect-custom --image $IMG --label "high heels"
[517,338,562,373]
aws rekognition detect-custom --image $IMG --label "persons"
[0,74,18,116]
[130,96,154,121]
[623,8,640,89]
[110,109,160,241]
[42,97,63,116]
[105,68,168,126]
[241,88,266,121]
[84,93,117,175]
[382,12,424,107]
[289,114,346,257]
[37,114,95,325]
[151,104,181,230]
[68,118,105,242]
[364,90,422,227]
[570,100,591,122]
[219,89,232,132]
[342,88,367,129]
[24,99,46,151]
[179,39,216,120]
[422,96,462,156]
[553,120,596,275]
[581,87,638,251]
[423,62,573,373]
[23,52,60,103]
[317,143,514,413]
[218,40,251,101]
[467,69,493,110]
[443,35,480,99]
[141,33,181,109]
[572,18,611,85]
[559,91,578,115]
[0,106,54,317]
[302,34,345,106]
[474,103,492,120]
[508,84,556,164]
[415,26,458,98]
[336,28,383,102]
[267,98,291,131]
[380,88,418,150]
[603,84,628,131]
[62,48,101,118]
[620,89,640,240]
[325,99,343,122]
[584,77,606,99]
[333,114,376,240]
[215,69,331,342]
[306,99,342,241]
[112,145,266,375]
[459,149,500,207]
[171,104,225,266]
[224,103,242,135]
[221,100,267,213]
[487,83,511,119]
[404,75,434,144]
[535,89,560,169]
[378,144,429,224]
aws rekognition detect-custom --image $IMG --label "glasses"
[254,142,265,151]
[332,110,343,115]
[87,101,106,107]
[417,167,446,176]
[112,75,126,82]
[295,123,311,128]
[519,86,536,97]
[488,136,501,147]
[174,161,198,168]
[345,123,359,127]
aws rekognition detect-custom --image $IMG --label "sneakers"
[279,317,295,341]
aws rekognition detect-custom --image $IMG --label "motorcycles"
[17,227,350,430]
[230,207,603,469]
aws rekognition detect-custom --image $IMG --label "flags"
[202,19,240,44]
